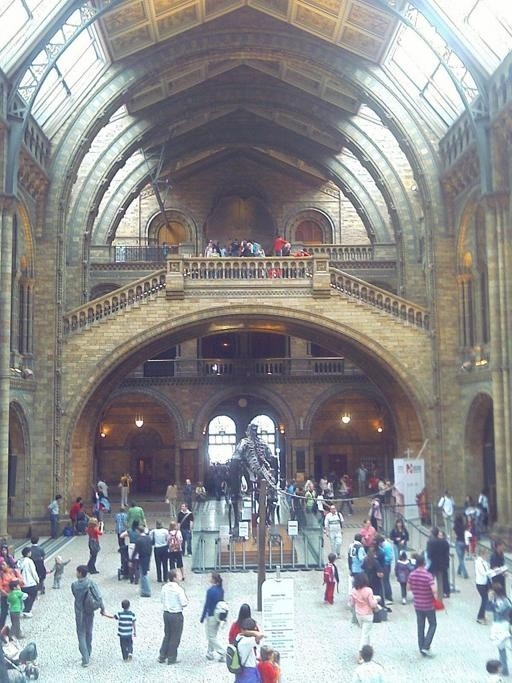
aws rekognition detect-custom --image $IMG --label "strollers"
[94,497,111,514]
[118,545,129,580]
[1,625,39,682]
[74,511,88,534]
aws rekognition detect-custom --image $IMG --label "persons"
[0,457,512,683]
[162,236,311,278]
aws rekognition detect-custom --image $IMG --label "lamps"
[12,366,34,381]
[461,358,475,373]
[134,413,144,429]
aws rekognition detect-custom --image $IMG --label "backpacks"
[227,637,252,673]
[168,529,180,549]
[324,568,333,583]
[214,601,229,622]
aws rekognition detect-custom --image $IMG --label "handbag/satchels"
[151,540,155,545]
[83,592,100,614]
[89,540,100,552]
[431,596,444,611]
